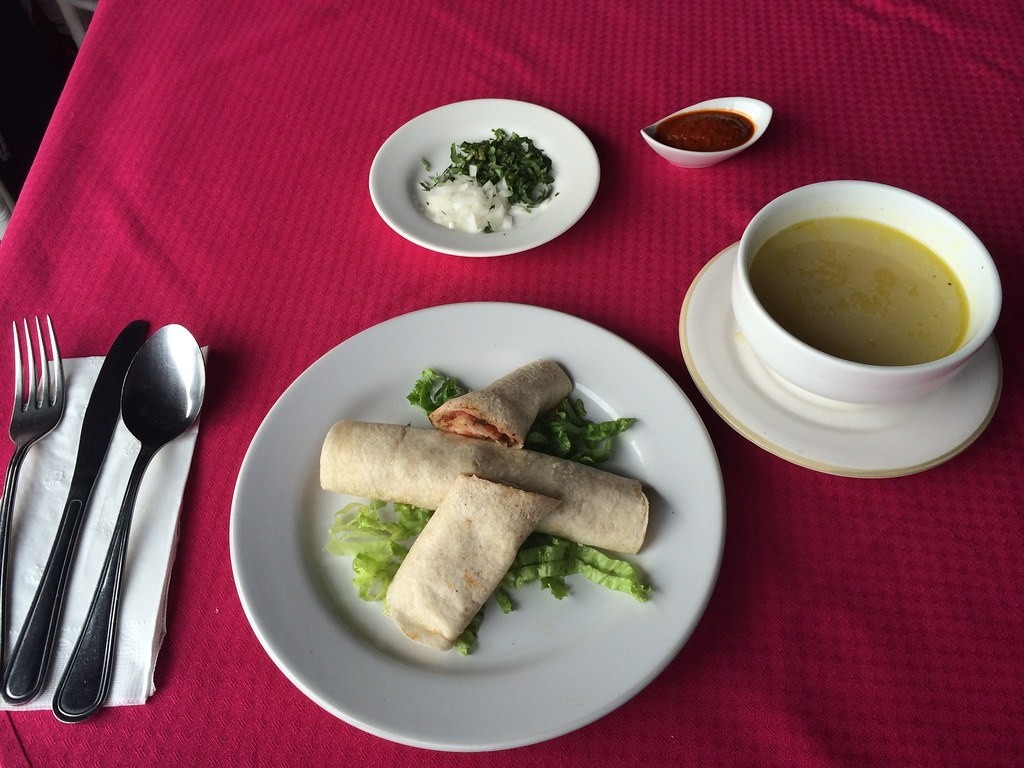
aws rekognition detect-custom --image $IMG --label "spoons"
[51,322,205,725]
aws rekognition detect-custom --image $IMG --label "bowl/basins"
[733,179,1002,407]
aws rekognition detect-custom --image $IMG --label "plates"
[228,301,724,751]
[679,238,1000,478]
[641,96,774,168]
[369,98,599,258]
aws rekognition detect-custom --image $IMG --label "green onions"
[419,127,559,235]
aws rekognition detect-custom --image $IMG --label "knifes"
[3,319,150,705]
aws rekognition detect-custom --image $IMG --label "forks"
[1,314,67,699]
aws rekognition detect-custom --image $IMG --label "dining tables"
[0,0,1024,768]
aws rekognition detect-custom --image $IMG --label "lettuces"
[325,368,650,655]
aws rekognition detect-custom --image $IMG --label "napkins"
[0,343,213,717]
